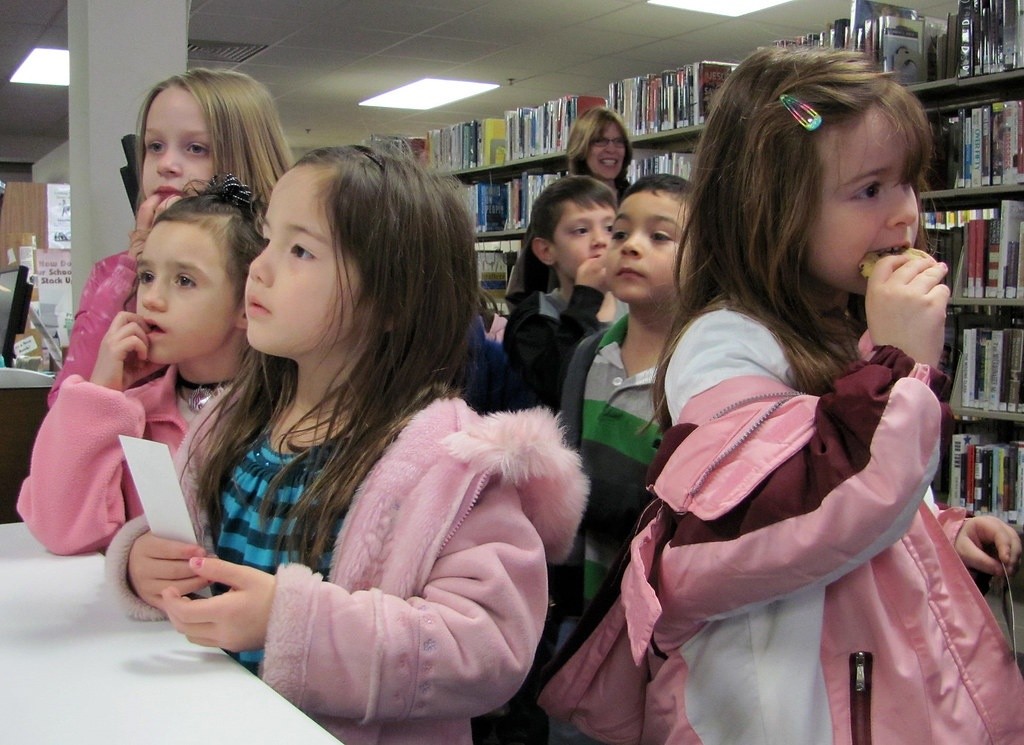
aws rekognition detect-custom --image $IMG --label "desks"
[1,368,348,745]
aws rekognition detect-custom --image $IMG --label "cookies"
[859,248,935,283]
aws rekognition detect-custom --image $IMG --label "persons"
[496,170,630,413]
[525,171,693,744]
[43,66,299,409]
[12,165,270,561]
[103,139,590,745]
[500,102,636,310]
[532,39,1024,743]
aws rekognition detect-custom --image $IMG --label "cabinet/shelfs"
[440,67,1023,659]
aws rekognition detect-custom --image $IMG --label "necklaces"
[175,369,241,414]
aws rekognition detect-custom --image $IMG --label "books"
[362,58,738,298]
[777,0,1024,526]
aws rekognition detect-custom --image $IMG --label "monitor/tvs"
[0,265,34,369]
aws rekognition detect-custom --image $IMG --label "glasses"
[593,136,625,147]
